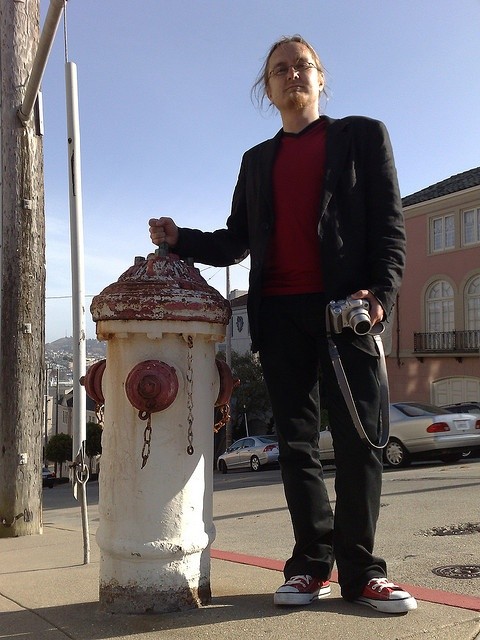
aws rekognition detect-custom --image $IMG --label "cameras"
[328,296,386,336]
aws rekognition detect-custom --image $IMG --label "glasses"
[267,61,320,79]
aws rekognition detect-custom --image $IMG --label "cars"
[318,400,480,474]
[41,468,57,489]
[216,433,285,474]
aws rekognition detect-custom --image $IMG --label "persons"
[149,33,417,614]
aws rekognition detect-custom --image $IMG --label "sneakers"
[272,573,331,607]
[353,571,415,615]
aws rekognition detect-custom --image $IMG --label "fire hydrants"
[77,244,241,613]
